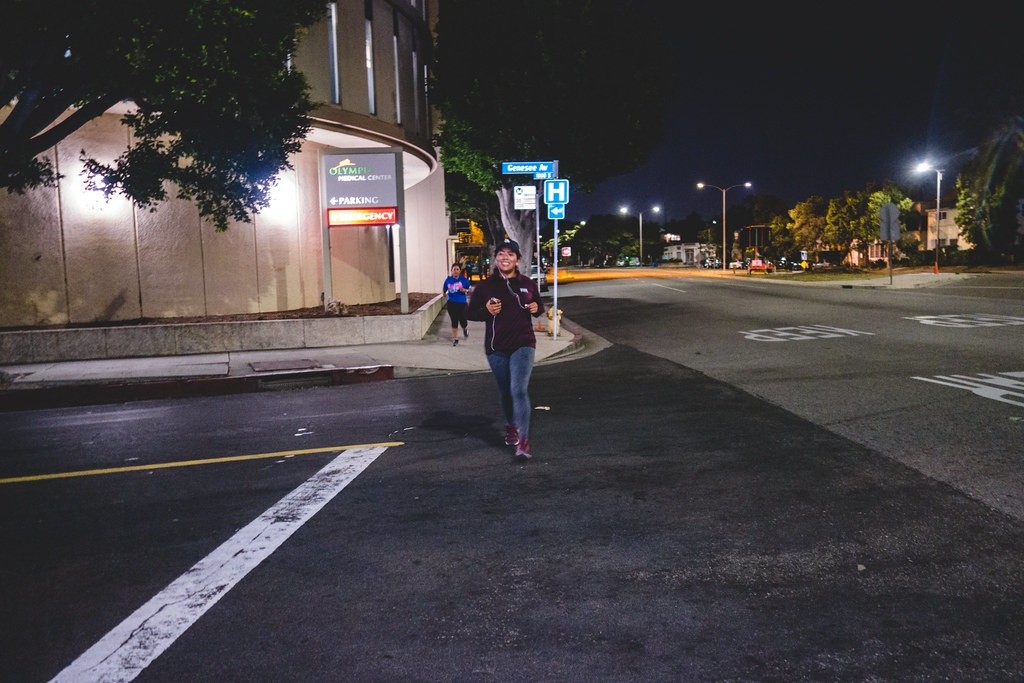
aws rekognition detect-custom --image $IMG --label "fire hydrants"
[547,306,563,337]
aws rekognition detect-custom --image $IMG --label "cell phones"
[490,297,498,304]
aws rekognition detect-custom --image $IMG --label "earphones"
[525,304,528,308]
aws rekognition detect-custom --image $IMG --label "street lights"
[914,163,942,272]
[619,207,661,266]
[696,182,753,269]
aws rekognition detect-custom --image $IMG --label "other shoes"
[452,338,459,346]
[463,330,469,338]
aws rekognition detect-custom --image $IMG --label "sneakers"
[515,437,532,460]
[505,424,520,446]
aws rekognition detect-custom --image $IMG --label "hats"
[495,239,521,256]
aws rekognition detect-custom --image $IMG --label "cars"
[529,258,550,292]
[604,256,640,268]
[701,256,801,274]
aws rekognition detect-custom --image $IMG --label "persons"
[478,261,488,281]
[442,264,472,346]
[466,239,543,458]
[465,260,472,281]
